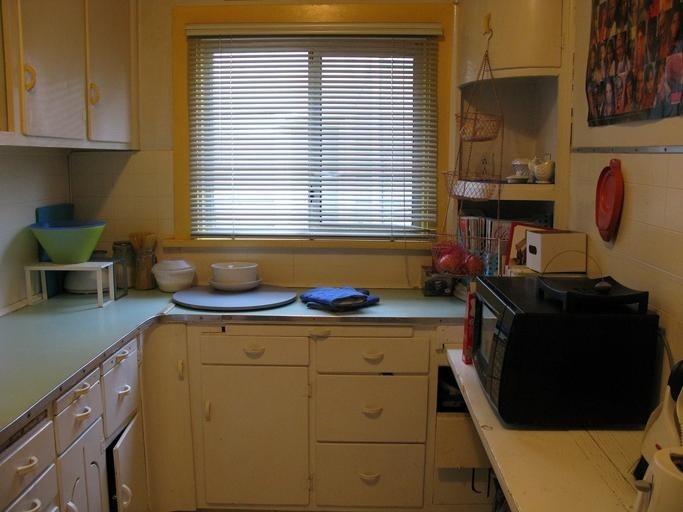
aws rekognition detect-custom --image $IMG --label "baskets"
[445,171,497,202]
[455,112,502,141]
[431,234,498,276]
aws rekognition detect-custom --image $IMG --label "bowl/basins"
[31,217,106,262]
[153,259,196,292]
[208,261,262,291]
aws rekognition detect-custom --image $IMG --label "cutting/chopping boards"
[173,287,297,311]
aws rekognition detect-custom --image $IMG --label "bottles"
[113,241,157,291]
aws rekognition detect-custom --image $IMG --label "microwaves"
[471,275,659,431]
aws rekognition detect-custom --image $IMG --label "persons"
[586,0,682,119]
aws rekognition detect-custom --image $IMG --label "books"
[460,216,529,254]
[454,284,467,301]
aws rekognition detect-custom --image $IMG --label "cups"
[512,159,554,185]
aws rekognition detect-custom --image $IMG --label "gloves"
[299,286,380,312]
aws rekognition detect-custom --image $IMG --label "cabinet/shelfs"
[0,0,132,151]
[450,1,573,303]
[139,324,200,510]
[432,320,500,509]
[1,412,61,512]
[53,365,103,512]
[313,325,432,510]
[196,328,312,510]
[101,337,148,511]
